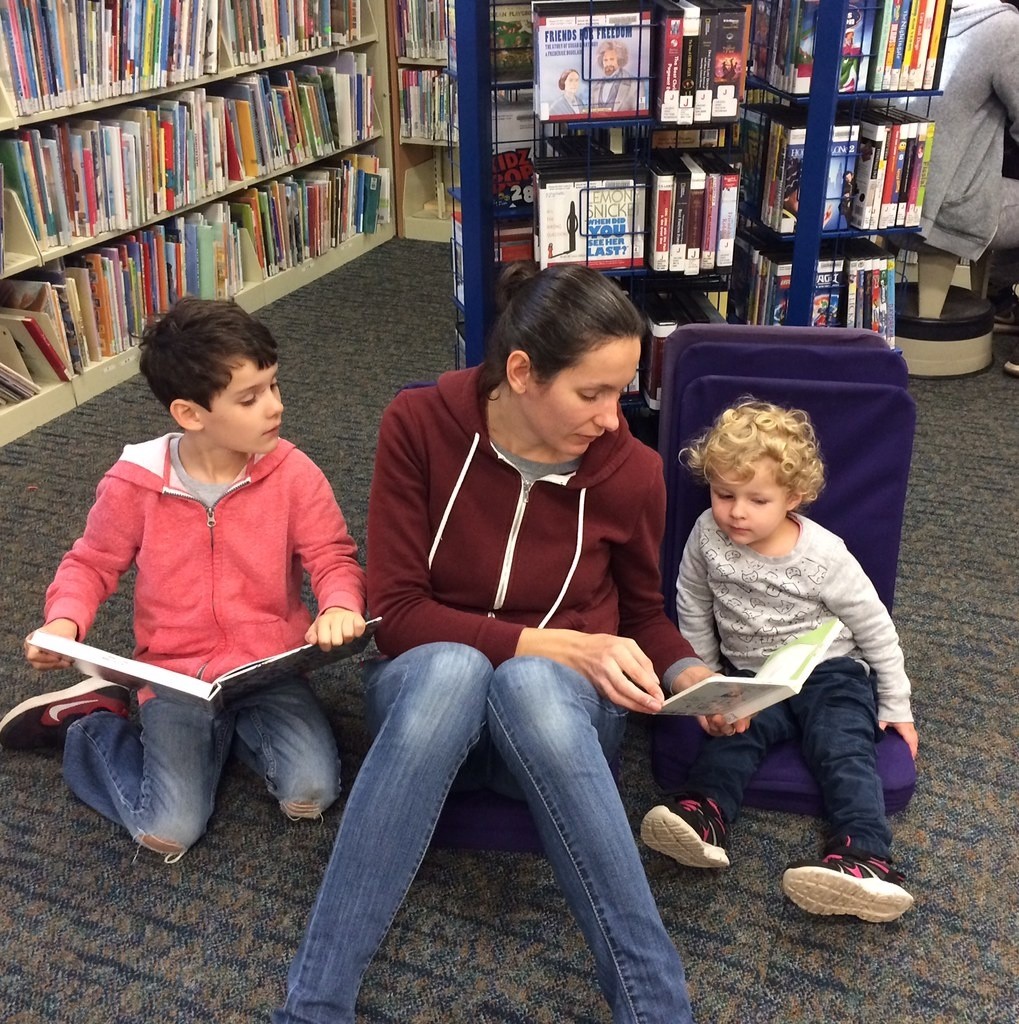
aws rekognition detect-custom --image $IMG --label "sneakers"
[0,674,130,748]
[641,794,731,870]
[1004,351,1019,375]
[990,293,1019,332]
[782,838,913,923]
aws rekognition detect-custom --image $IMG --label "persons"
[640,392,921,920]
[274,259,750,1023]
[916,0,1018,382]
[0,293,367,865]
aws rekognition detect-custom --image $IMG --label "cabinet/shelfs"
[0,0,957,449]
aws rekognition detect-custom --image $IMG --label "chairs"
[654,320,916,814]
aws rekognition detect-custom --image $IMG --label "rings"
[726,728,736,737]
